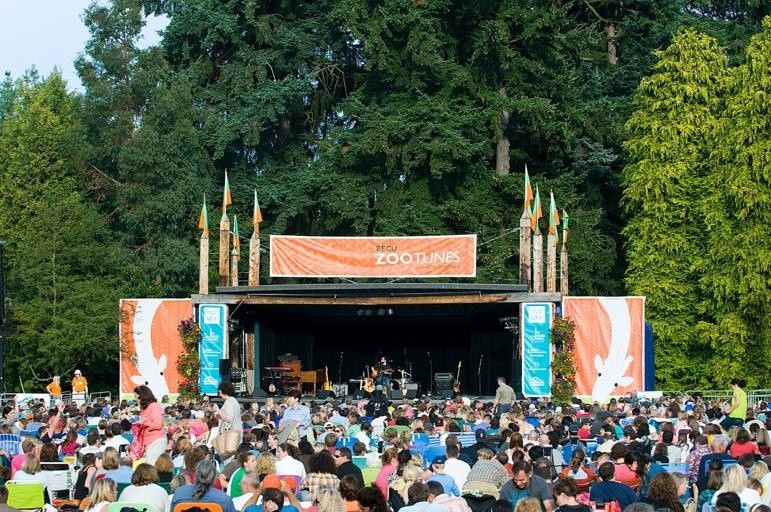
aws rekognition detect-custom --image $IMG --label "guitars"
[323,366,332,390]
[362,365,375,393]
[370,368,394,379]
[453,360,463,392]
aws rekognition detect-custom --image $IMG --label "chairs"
[2,392,771,512]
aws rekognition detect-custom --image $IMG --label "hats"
[324,421,334,427]
[73,370,80,375]
[433,456,445,464]
[476,428,487,443]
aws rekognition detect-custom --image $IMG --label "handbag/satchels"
[211,429,241,459]
[128,424,146,459]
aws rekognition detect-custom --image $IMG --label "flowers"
[548,314,578,393]
[177,317,202,391]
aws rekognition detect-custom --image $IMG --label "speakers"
[353,390,369,399]
[442,390,453,399]
[318,390,336,399]
[391,390,403,399]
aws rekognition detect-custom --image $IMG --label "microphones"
[481,355,483,362]
[427,352,431,358]
[341,352,344,359]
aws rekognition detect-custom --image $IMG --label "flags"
[233,218,241,261]
[225,176,232,205]
[198,204,208,231]
[251,191,262,236]
[524,168,570,244]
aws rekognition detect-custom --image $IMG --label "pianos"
[263,376,300,380]
[287,370,326,398]
